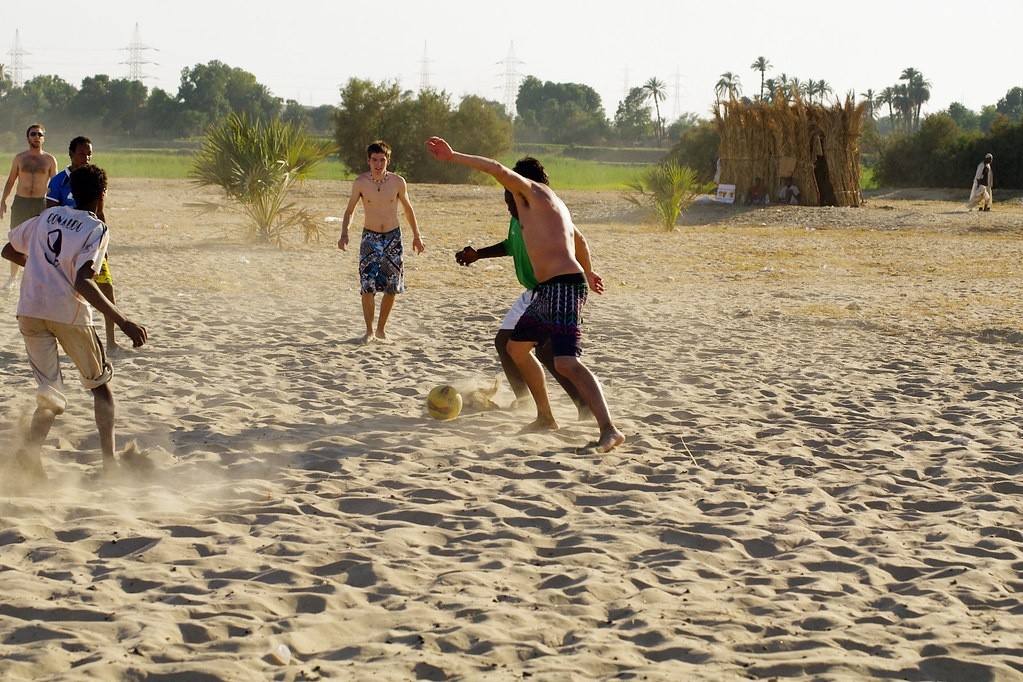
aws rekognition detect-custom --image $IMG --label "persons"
[337,141,426,347]
[966,152,994,212]
[44,136,139,358]
[1,164,148,485]
[423,134,627,453]
[454,182,598,424]
[1,123,59,292]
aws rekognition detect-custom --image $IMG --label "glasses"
[28,132,44,137]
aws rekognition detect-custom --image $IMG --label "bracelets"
[341,234,348,238]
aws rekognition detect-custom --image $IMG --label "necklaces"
[368,169,388,193]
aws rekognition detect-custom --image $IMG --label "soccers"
[426,384,463,421]
